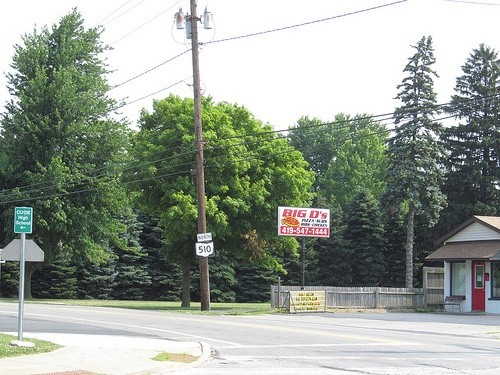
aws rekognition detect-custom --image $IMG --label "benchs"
[436,296,463,312]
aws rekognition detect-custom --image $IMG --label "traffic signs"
[13,206,33,234]
